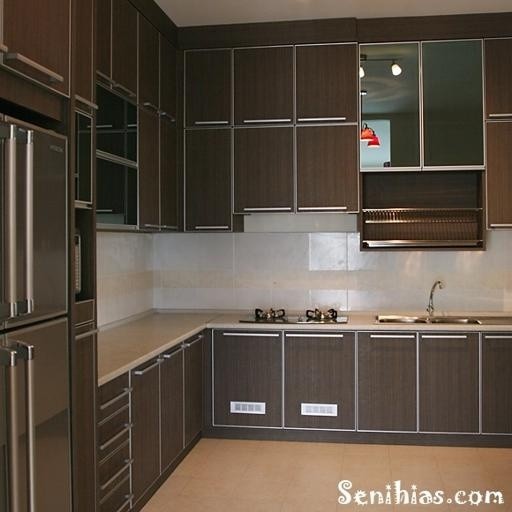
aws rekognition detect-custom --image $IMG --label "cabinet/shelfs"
[211,328,356,431]
[232,41,359,125]
[80,131,126,215]
[138,8,180,120]
[233,125,359,215]
[1,0,69,128]
[479,36,512,231]
[78,98,137,132]
[180,41,244,234]
[129,351,161,507]
[160,332,204,474]
[480,332,510,434]
[359,40,483,171]
[356,332,480,434]
[138,102,180,234]
[94,0,138,98]
[69,0,93,113]
[98,367,131,510]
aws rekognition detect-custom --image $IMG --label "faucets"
[424,280,445,322]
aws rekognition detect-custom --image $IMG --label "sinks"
[371,315,483,326]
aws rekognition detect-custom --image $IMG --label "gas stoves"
[239,305,350,325]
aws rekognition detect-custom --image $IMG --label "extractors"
[233,211,361,235]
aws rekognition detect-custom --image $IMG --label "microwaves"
[73,226,83,294]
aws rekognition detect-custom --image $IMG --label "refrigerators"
[0,111,77,508]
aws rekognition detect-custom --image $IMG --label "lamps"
[358,58,402,78]
[360,90,380,148]
